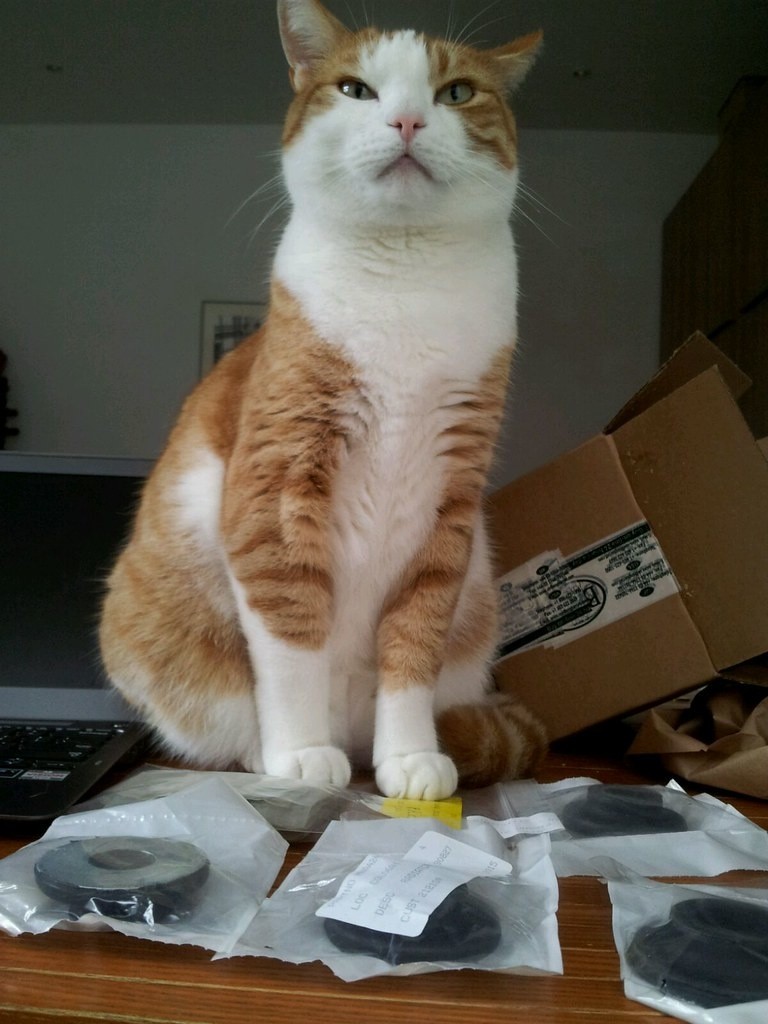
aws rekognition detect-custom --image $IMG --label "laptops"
[0,451,162,843]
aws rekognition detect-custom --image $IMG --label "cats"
[101,1,545,800]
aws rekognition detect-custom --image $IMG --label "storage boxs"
[480,330,768,745]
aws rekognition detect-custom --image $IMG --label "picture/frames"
[198,300,269,386]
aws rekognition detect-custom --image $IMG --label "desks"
[0,738,768,1024]
